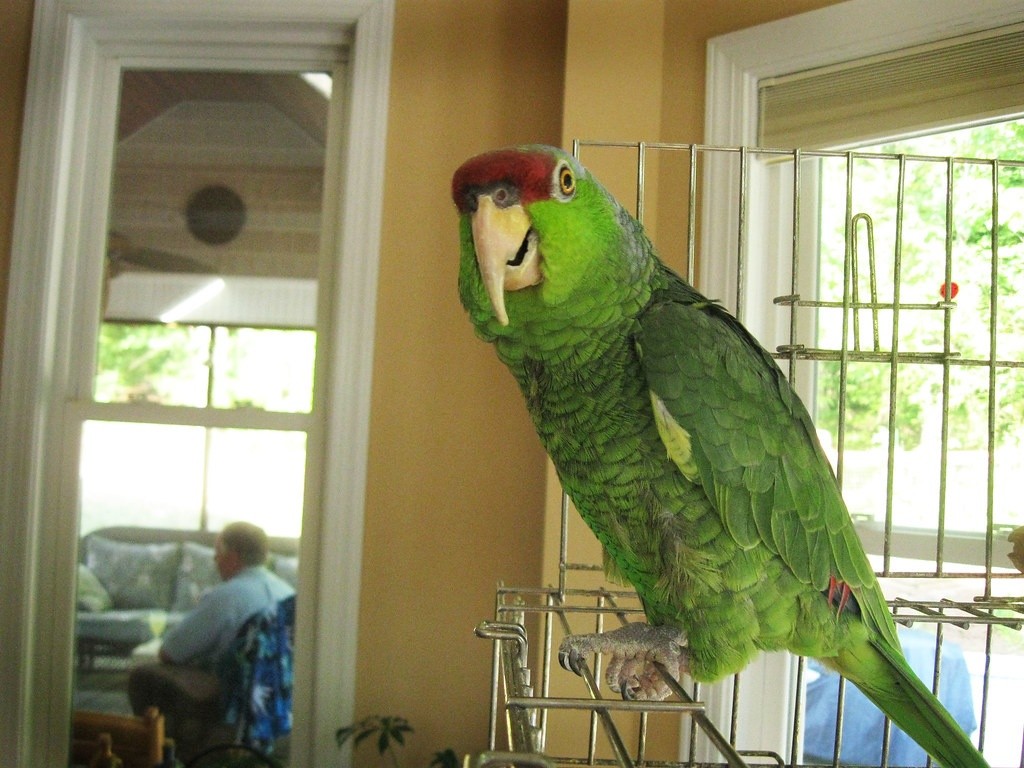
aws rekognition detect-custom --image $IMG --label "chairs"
[129,596,295,767]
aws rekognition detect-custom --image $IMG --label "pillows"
[86,533,181,609]
[274,553,298,586]
[77,562,113,614]
[168,542,272,615]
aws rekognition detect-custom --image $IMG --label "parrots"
[451,143,992,768]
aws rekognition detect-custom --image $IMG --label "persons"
[129,522,295,767]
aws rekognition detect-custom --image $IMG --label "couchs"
[74,526,300,670]
